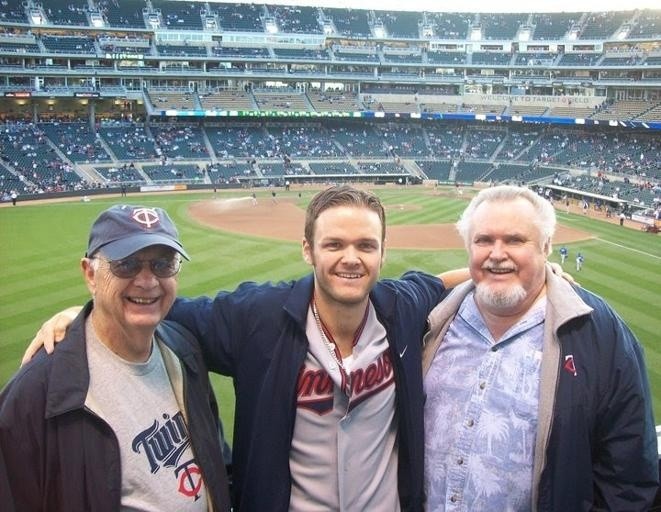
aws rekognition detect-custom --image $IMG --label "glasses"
[92,255,182,280]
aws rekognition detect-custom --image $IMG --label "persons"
[420,185,660,512]
[575,252,585,271]
[0,202,234,511]
[559,245,568,264]
[1,1,660,227]
[16,184,473,511]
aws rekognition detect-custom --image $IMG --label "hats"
[86,204,192,267]
